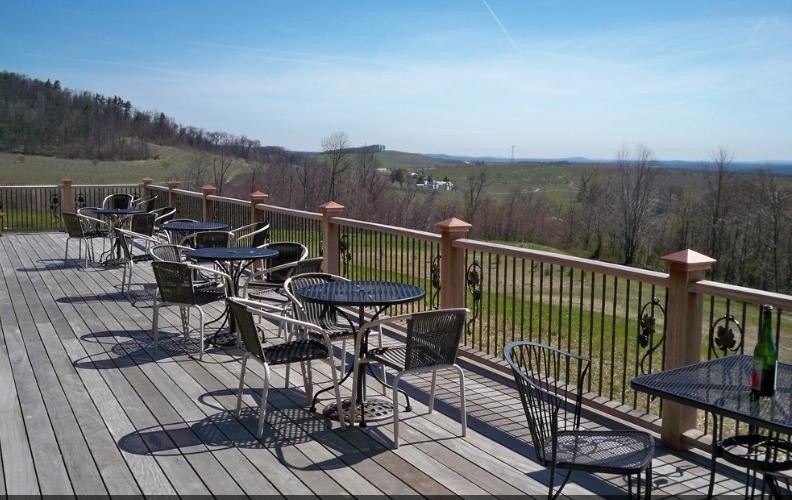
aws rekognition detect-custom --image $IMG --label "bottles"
[752,303,779,396]
